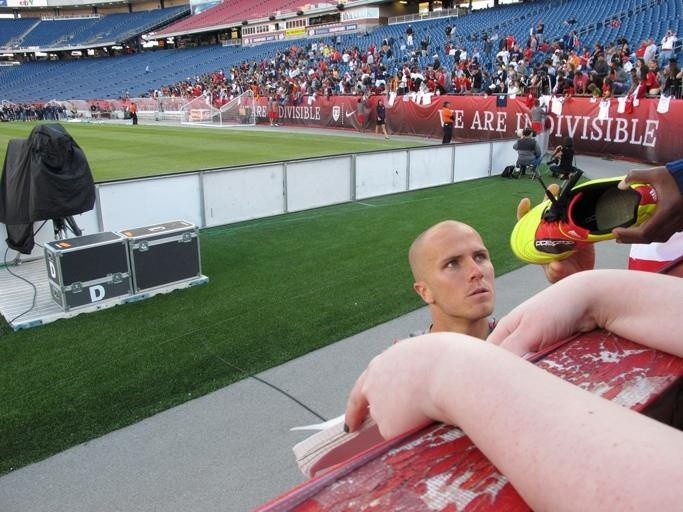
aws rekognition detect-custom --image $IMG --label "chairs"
[517,153,548,182]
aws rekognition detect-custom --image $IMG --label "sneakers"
[511,174,659,265]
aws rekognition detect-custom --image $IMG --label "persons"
[512,128,543,173]
[549,135,576,180]
[442,100,455,144]
[408,184,595,340]
[344,268,683,512]
[1,103,77,122]
[612,159,683,244]
[89,95,138,125]
[375,99,390,139]
[146,23,682,133]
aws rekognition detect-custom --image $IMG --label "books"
[288,407,384,480]
